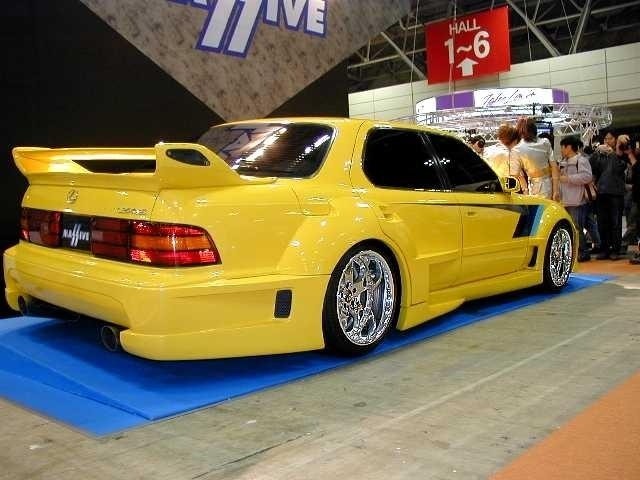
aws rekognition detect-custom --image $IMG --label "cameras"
[478,141,484,148]
[619,138,636,151]
[594,142,608,151]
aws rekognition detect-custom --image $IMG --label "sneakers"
[578,249,640,264]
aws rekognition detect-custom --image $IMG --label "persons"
[463,116,640,266]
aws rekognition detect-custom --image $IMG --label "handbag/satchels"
[584,181,599,204]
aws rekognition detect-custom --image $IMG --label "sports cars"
[2,117,580,362]
